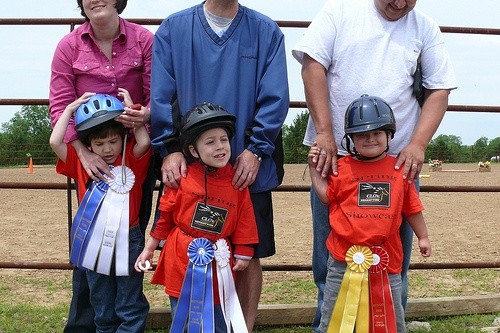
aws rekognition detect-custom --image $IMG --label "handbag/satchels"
[414,55,424,108]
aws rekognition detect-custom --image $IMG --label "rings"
[320,151,327,156]
[412,163,418,166]
[132,121,135,127]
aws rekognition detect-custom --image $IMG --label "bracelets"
[255,152,264,161]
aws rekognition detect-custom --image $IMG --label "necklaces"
[203,0,240,26]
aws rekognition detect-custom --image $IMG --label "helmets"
[74,94,126,132]
[176,102,237,161]
[344,95,396,137]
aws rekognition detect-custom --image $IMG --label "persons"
[149,0,302,332]
[134,102,259,333]
[292,0,457,332]
[307,94,432,333]
[50,87,152,332]
[49,0,154,333]
